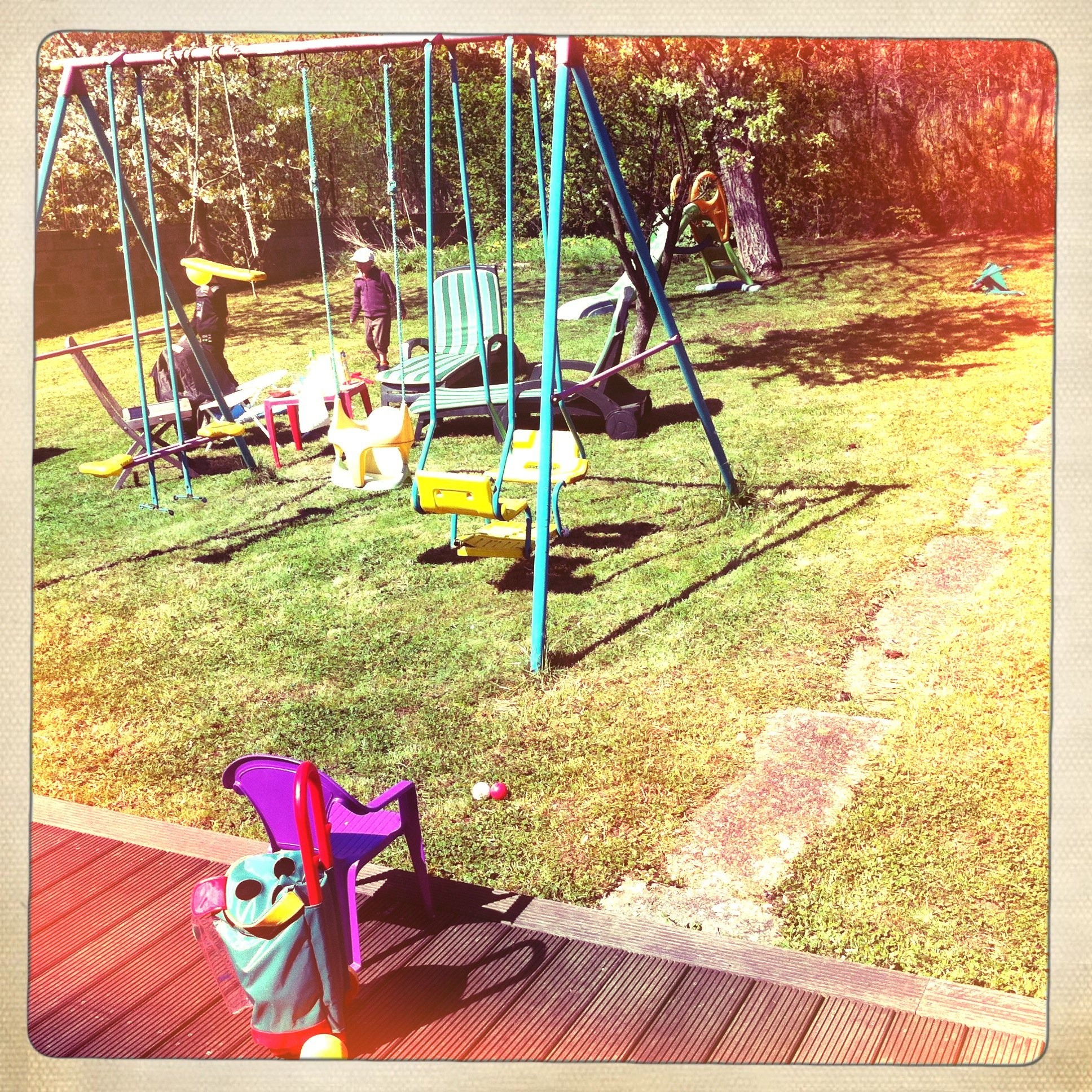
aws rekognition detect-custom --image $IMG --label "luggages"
[191,758,350,1058]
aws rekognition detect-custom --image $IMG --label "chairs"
[406,286,653,447]
[66,336,200,488]
[220,755,432,969]
[376,266,503,406]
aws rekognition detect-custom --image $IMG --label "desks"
[264,382,372,467]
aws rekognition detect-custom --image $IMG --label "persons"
[349,247,406,373]
[178,243,239,387]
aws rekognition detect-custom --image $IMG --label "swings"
[77,45,250,479]
[293,49,422,496]
[407,36,591,564]
[157,48,272,288]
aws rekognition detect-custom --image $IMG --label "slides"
[557,201,699,321]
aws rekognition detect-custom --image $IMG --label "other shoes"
[374,361,389,369]
[378,365,390,372]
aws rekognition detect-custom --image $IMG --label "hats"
[349,248,375,263]
